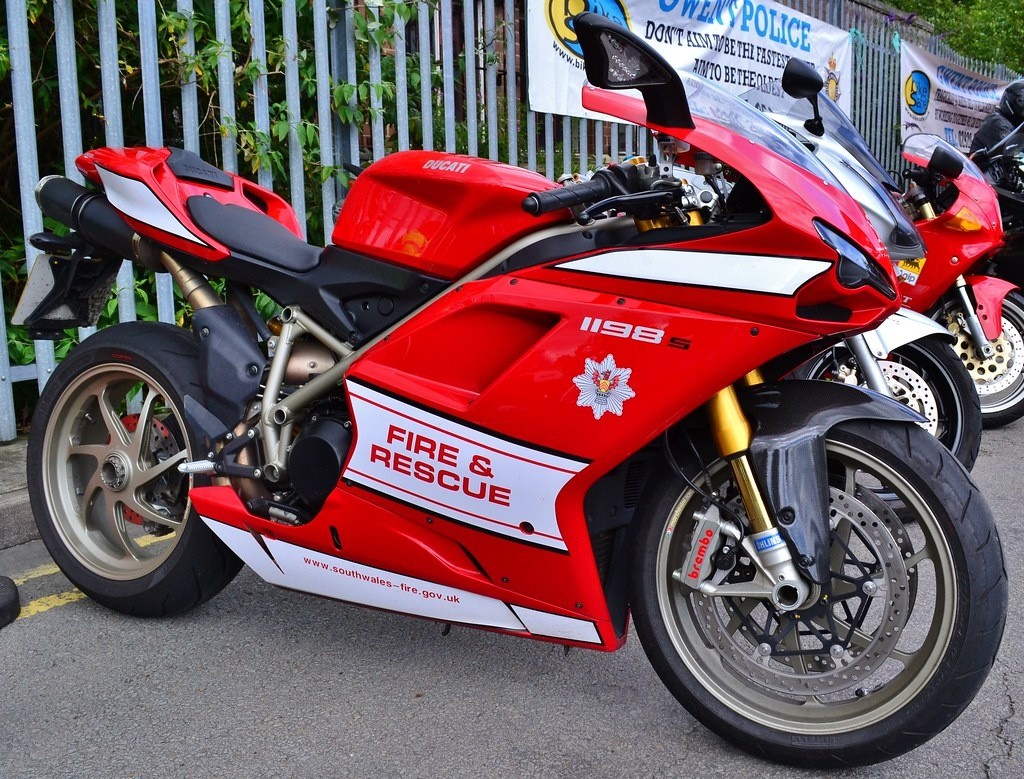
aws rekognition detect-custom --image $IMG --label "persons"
[969,79,1024,174]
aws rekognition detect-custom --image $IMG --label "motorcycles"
[732,57,983,524]
[8,13,1010,772]
[881,131,1023,430]
[967,140,1023,290]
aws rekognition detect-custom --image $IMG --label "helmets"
[1000,79,1024,130]
